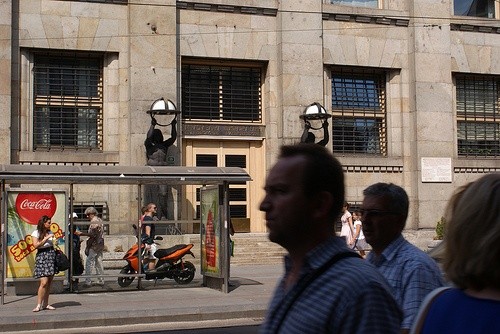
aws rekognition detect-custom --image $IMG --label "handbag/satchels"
[55,250,70,271]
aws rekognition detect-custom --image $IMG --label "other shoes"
[33,307,42,312]
[83,280,91,287]
[93,280,104,285]
[42,304,55,309]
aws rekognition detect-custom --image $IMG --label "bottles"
[52,236,57,248]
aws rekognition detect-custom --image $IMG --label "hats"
[69,212,78,218]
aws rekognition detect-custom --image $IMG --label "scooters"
[118,223,196,287]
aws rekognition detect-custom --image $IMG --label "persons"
[144,119,177,221]
[361,181,444,334]
[352,212,369,256]
[68,207,104,288]
[300,121,329,147]
[409,171,500,334]
[31,215,58,312]
[138,202,157,270]
[340,202,354,249]
[258,143,403,334]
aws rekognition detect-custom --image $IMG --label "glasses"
[154,207,157,209]
[87,214,90,216]
[354,208,398,218]
